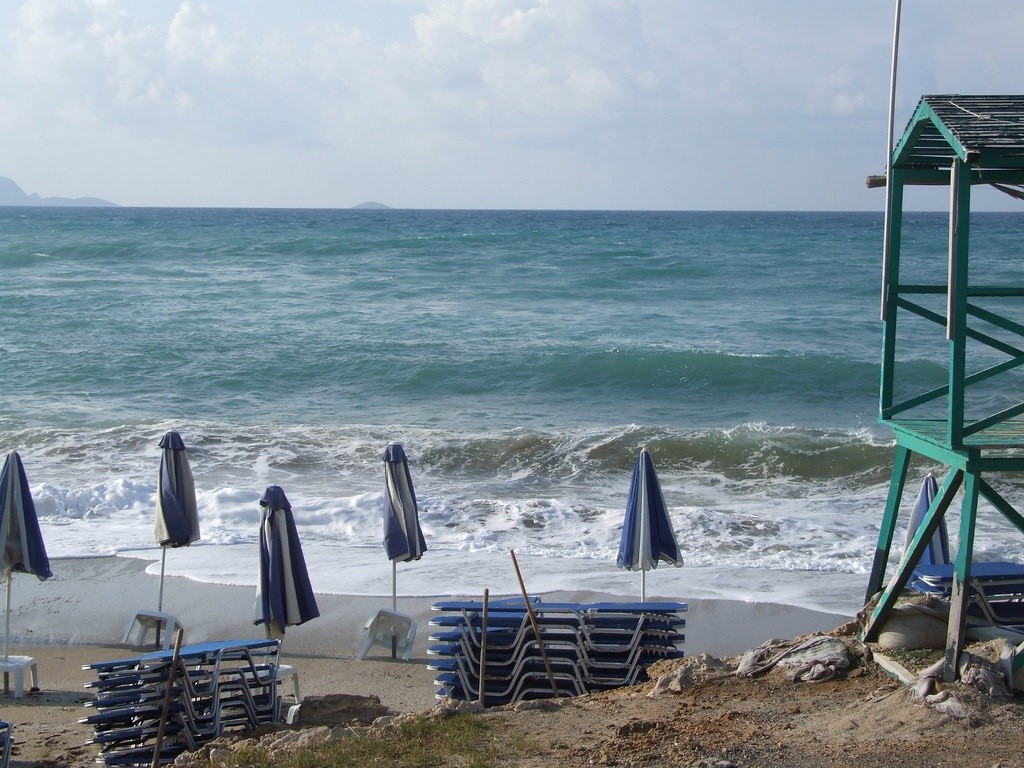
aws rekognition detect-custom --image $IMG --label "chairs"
[428,596,688,708]
[76,640,299,768]
[912,562,1024,632]
[0,721,15,768]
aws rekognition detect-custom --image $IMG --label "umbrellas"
[616,447,684,604]
[383,442,428,658]
[154,429,201,645]
[900,472,949,590]
[0,449,54,693]
[252,486,320,693]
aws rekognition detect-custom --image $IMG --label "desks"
[121,610,184,650]
[355,608,418,661]
[0,656,38,699]
